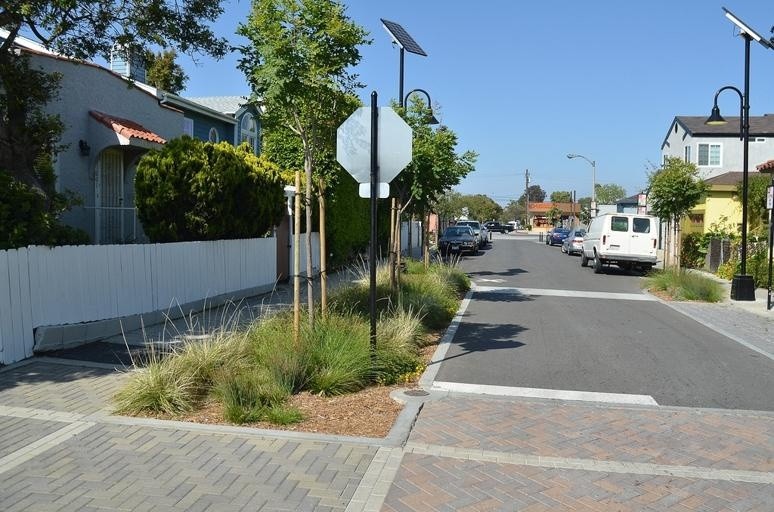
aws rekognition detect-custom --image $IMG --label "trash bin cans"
[528,226,531,231]
[539,233,543,242]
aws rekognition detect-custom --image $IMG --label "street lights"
[704,83,774,302]
[567,153,596,201]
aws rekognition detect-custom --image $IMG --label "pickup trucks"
[481,221,514,234]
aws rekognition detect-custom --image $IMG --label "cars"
[455,220,488,248]
[561,229,586,255]
[439,226,479,256]
[546,227,570,246]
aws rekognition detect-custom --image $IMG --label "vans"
[580,212,660,274]
